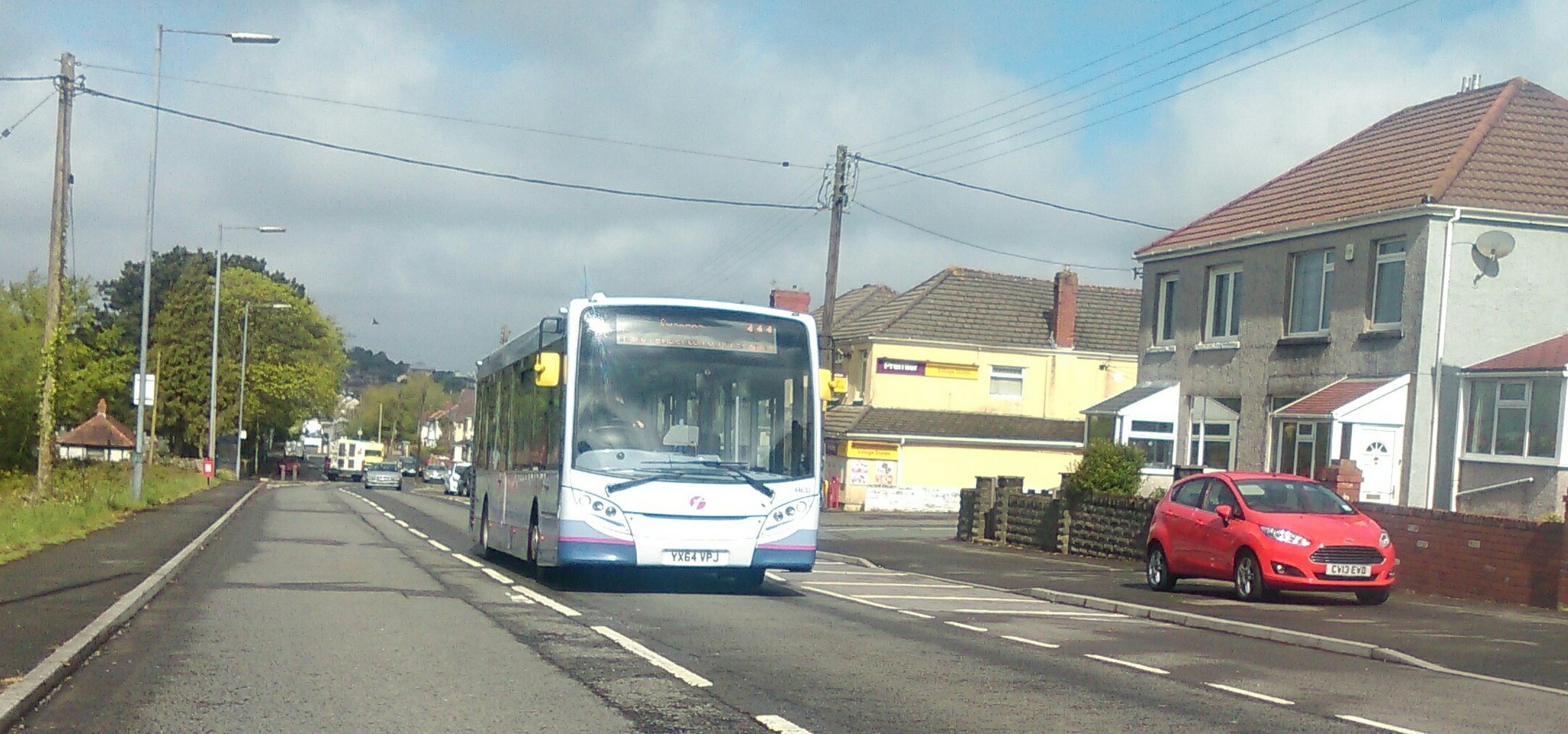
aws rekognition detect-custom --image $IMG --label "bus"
[467,291,837,591]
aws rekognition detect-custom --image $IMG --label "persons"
[579,373,644,446]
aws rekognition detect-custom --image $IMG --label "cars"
[457,466,472,498]
[421,465,450,483]
[324,456,331,475]
[444,462,470,496]
[1144,471,1396,606]
[397,456,417,477]
[364,463,402,491]
[277,457,301,476]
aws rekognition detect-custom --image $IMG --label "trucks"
[328,439,364,481]
[284,439,305,461]
[361,440,384,473]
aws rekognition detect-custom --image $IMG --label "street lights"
[236,302,293,479]
[135,24,279,501]
[208,223,284,478]
